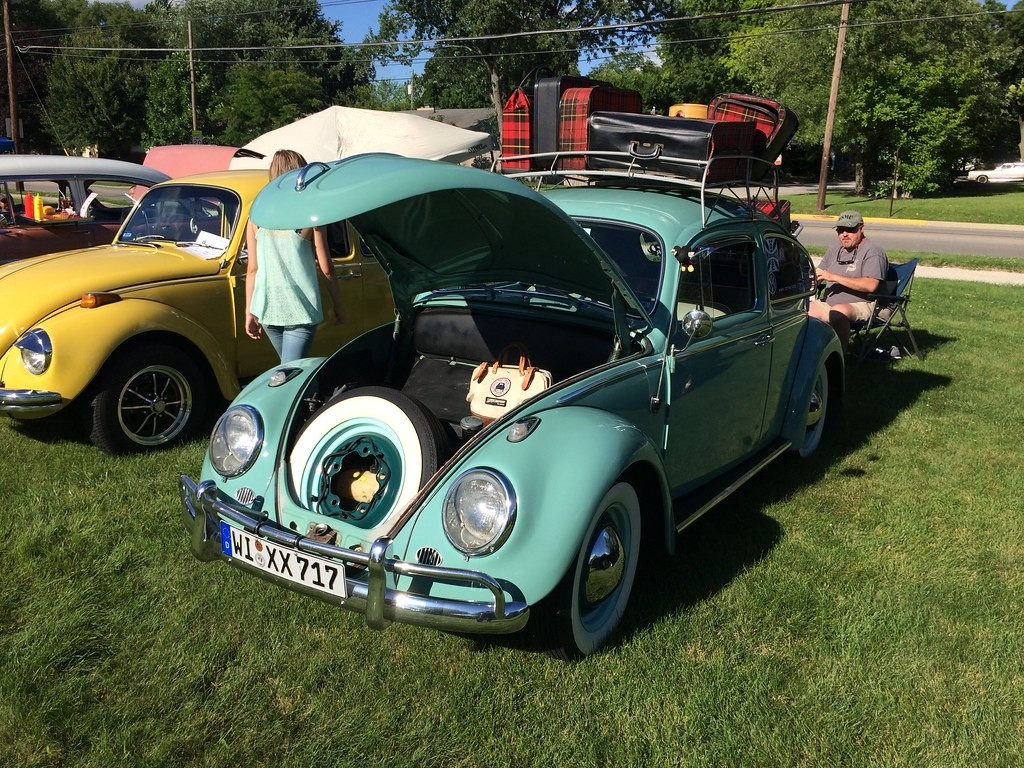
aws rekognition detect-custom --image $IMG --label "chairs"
[816,257,923,369]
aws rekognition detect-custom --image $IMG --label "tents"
[135,105,497,205]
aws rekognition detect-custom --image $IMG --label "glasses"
[836,227,860,232]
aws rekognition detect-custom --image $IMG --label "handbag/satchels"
[466,341,553,429]
[401,350,492,424]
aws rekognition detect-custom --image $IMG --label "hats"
[832,210,864,228]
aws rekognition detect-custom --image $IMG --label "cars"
[0,169,396,457]
[967,162,1024,184]
[176,151,848,662]
[0,154,173,265]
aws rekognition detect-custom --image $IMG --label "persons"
[808,210,888,356]
[243,148,346,365]
[0,192,15,213]
